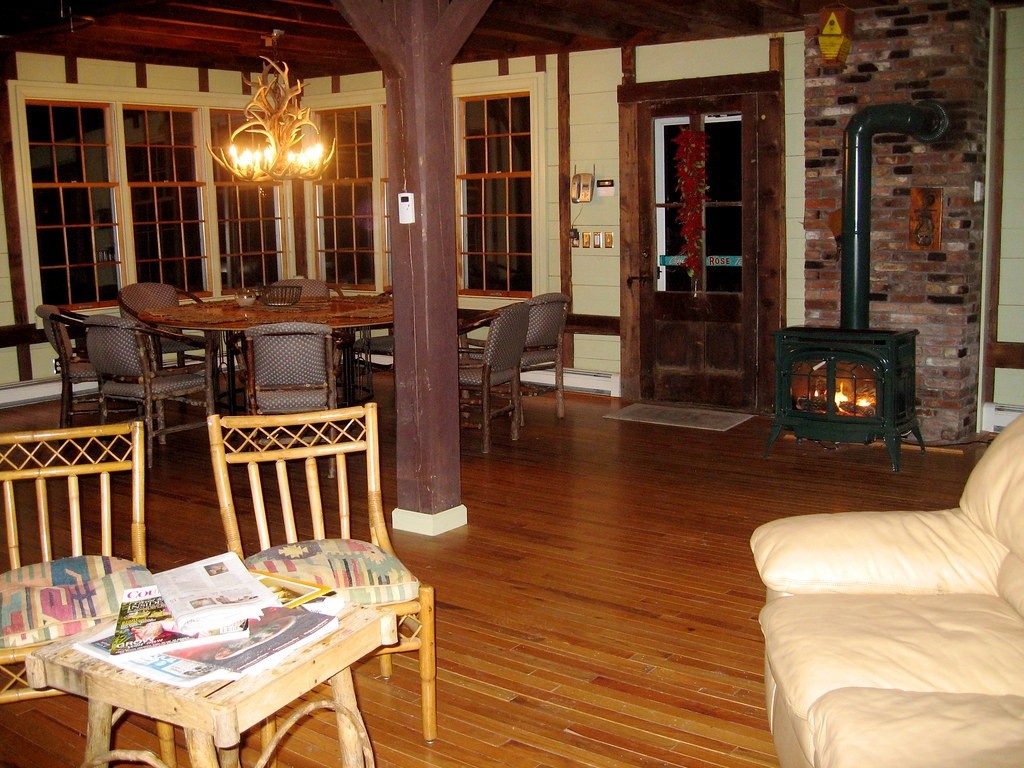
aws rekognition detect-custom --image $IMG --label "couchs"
[749,414,1024,768]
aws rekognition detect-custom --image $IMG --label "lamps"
[206,30,338,182]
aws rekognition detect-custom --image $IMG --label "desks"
[24,597,399,768]
[138,297,395,432]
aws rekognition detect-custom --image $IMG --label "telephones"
[570,173,595,203]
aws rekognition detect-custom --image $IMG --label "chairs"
[36,282,571,479]
[207,402,437,743]
[0,421,178,768]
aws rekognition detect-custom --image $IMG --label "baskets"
[260,286,302,306]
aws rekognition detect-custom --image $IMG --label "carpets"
[602,402,755,432]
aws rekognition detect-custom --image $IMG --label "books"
[73,550,343,689]
